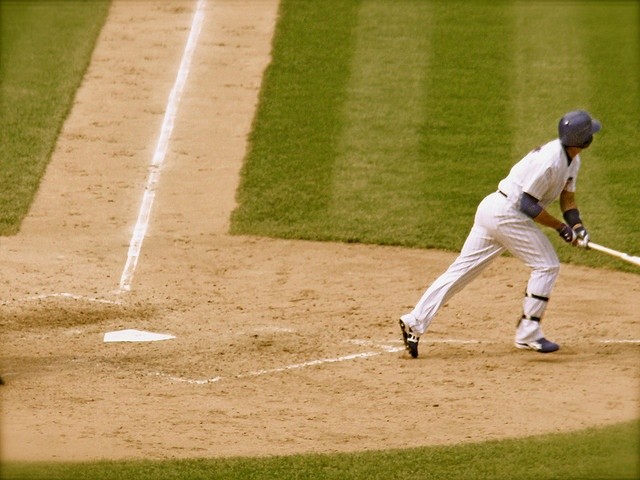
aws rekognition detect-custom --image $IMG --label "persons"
[398,110,603,359]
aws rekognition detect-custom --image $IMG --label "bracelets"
[563,207,582,228]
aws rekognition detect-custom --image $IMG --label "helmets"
[558,111,601,148]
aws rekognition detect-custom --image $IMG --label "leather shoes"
[500,191,508,197]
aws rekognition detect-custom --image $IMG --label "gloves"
[559,225,577,244]
[574,225,590,248]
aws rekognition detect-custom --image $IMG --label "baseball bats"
[577,239,640,266]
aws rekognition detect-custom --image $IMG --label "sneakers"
[399,316,420,358]
[514,337,559,353]
